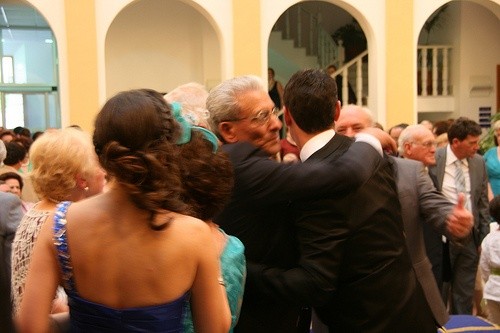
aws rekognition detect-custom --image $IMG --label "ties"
[453,160,466,193]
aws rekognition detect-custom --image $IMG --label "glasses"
[257,106,280,124]
[426,141,437,147]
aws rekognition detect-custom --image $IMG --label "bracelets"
[218,280,225,286]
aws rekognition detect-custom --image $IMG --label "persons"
[18,89,232,333]
[0,64,500,333]
